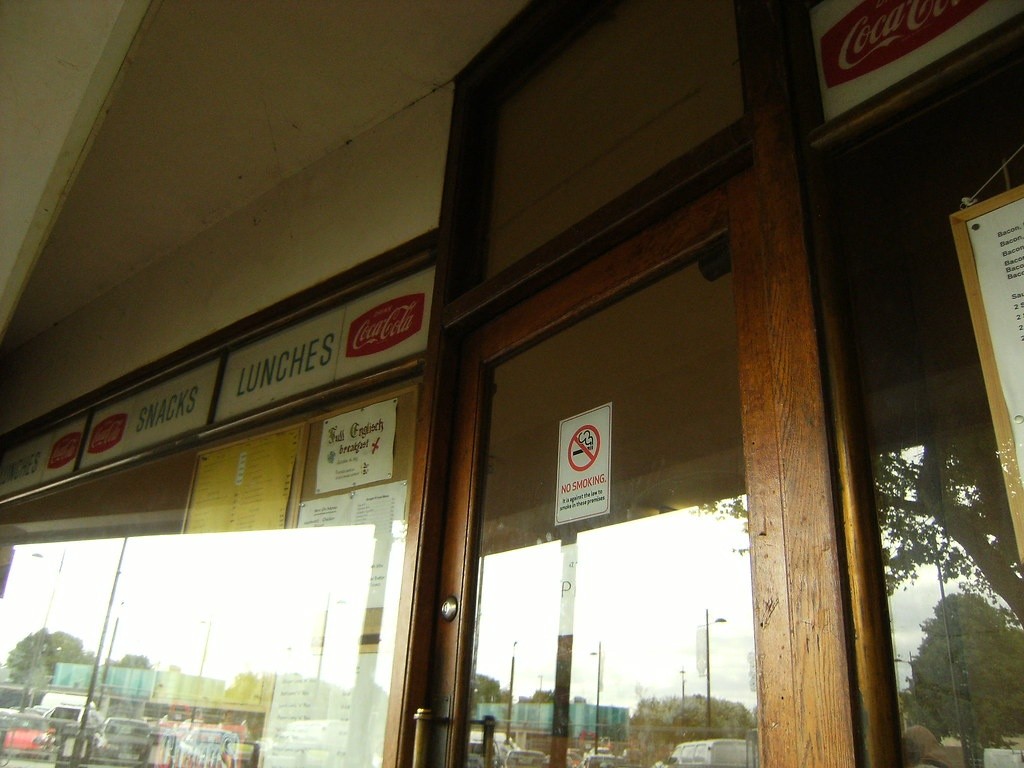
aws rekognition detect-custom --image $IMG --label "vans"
[469,730,519,753]
[652,739,759,768]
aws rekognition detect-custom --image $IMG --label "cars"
[178,728,240,768]
[503,750,547,768]
[41,704,96,728]
[0,708,60,768]
[568,747,642,768]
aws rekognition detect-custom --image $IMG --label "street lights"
[591,641,601,754]
[706,610,727,734]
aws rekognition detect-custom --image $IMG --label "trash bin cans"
[54,730,93,768]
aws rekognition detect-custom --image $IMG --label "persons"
[902,725,953,768]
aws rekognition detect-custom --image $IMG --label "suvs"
[467,741,504,768]
[92,717,152,763]
